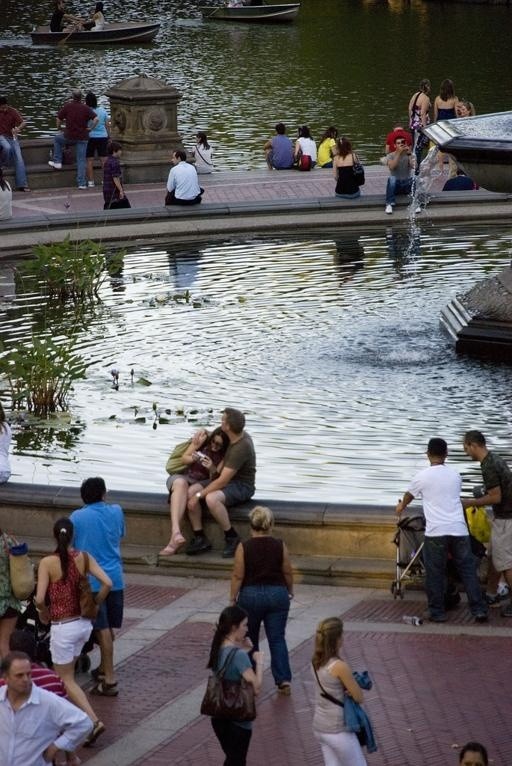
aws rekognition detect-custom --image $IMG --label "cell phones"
[197,452,205,464]
[401,140,405,146]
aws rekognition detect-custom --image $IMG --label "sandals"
[159,531,186,555]
[84,719,105,746]
[90,667,105,682]
[87,680,121,696]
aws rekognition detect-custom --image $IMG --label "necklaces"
[225,635,237,646]
[430,462,444,465]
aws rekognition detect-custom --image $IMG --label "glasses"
[210,439,223,447]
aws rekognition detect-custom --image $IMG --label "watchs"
[194,492,203,500]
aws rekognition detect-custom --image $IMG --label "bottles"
[402,615,424,627]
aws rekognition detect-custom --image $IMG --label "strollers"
[391,510,428,599]
[13,597,96,671]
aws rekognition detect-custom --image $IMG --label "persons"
[231,506,297,693]
[241,0,263,6]
[103,143,132,210]
[461,429,511,617]
[50,0,86,33]
[0,399,13,483]
[103,251,127,305]
[192,133,213,174]
[333,137,361,199]
[331,238,366,288]
[157,424,228,556]
[76,2,106,32]
[0,265,20,318]
[458,742,489,765]
[48,89,98,189]
[394,436,489,624]
[205,604,265,766]
[384,78,479,213]
[1,166,12,216]
[293,125,317,168]
[228,0,247,7]
[185,407,257,557]
[264,123,295,170]
[385,234,422,282]
[310,616,370,765]
[166,248,204,296]
[166,150,205,206]
[1,96,31,192]
[84,92,110,188]
[0,477,127,764]
[318,127,338,168]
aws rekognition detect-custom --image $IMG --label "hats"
[386,130,412,152]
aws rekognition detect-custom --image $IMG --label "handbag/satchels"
[8,543,35,600]
[166,439,193,475]
[293,155,312,173]
[352,163,365,186]
[80,580,100,619]
[201,676,256,719]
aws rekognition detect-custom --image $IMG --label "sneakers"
[188,536,212,552]
[428,607,448,622]
[16,187,31,192]
[48,161,61,168]
[79,185,87,190]
[386,204,393,213]
[415,207,422,214]
[503,605,512,616]
[475,608,487,621]
[490,593,512,608]
[222,534,241,558]
[88,181,95,187]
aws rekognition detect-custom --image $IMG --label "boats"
[200,2,306,24]
[30,19,164,48]
